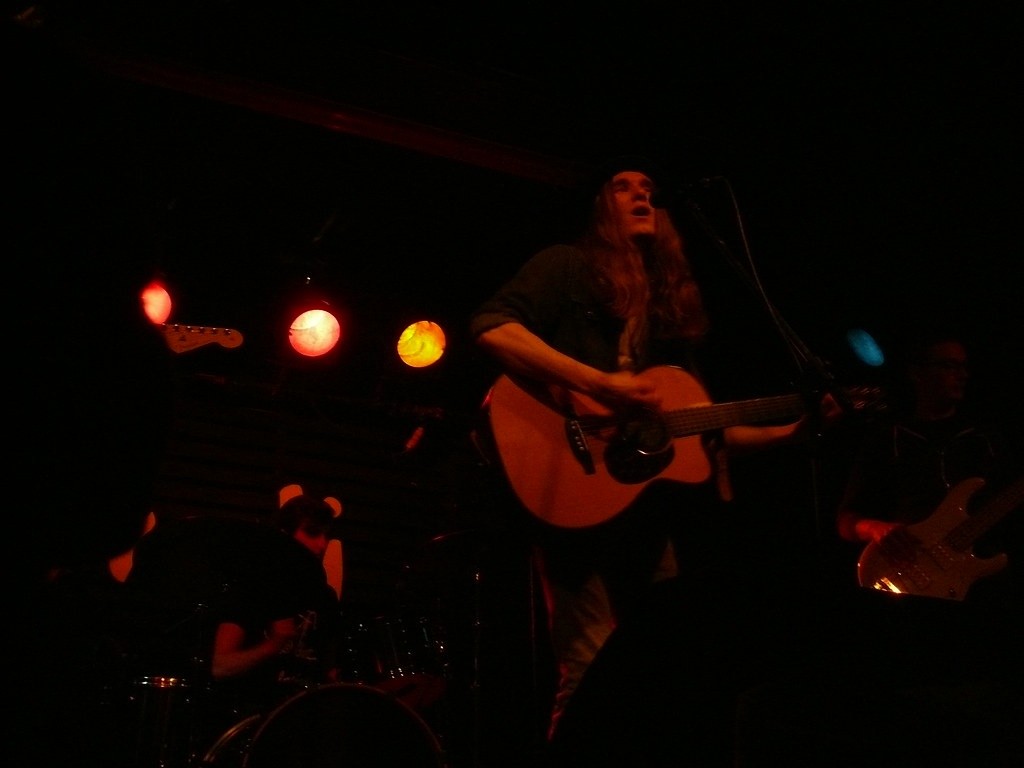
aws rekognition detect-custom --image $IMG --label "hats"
[576,150,662,228]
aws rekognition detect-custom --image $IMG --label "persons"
[213,481,339,721]
[470,168,844,742]
[837,317,1024,700]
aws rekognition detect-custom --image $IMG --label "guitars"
[473,348,882,538]
[842,476,1024,605]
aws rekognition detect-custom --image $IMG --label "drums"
[241,673,470,768]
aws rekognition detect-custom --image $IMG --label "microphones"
[650,174,725,208]
[402,422,428,452]
